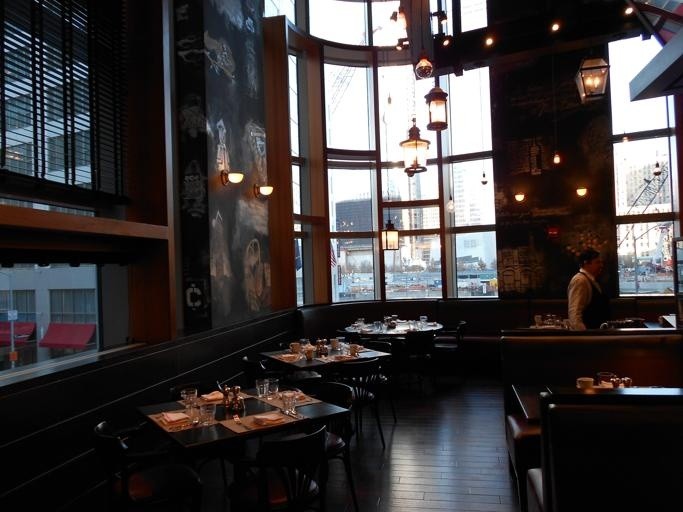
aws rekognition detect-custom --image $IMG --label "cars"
[399,283,425,292]
[343,281,372,296]
[636,265,647,276]
[471,285,496,296]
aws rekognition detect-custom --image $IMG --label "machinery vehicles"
[650,223,672,272]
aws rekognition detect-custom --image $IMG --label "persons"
[566,249,607,331]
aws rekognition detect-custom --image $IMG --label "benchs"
[298,298,683,346]
[499,333,683,504]
[525,386,683,512]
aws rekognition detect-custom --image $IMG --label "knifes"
[168,417,199,430]
[279,410,297,419]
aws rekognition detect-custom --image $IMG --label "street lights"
[633,222,661,295]
[7,334,29,370]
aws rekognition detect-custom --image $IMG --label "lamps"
[221,170,245,187]
[378,0,446,251]
[253,183,274,198]
[573,0,610,105]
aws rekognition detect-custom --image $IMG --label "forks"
[320,355,334,362]
[231,415,252,431]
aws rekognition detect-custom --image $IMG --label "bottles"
[222,385,245,413]
[315,339,327,354]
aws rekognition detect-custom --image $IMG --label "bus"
[457,273,489,288]
[635,256,659,276]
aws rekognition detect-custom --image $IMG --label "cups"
[355,314,427,331]
[254,378,280,400]
[180,387,216,426]
[575,371,631,390]
[281,389,297,414]
[330,336,359,357]
[534,314,556,327]
[288,338,313,360]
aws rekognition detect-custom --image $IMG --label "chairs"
[334,318,468,380]
[92,381,363,512]
[241,334,397,451]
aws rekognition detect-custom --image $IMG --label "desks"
[511,383,683,423]
